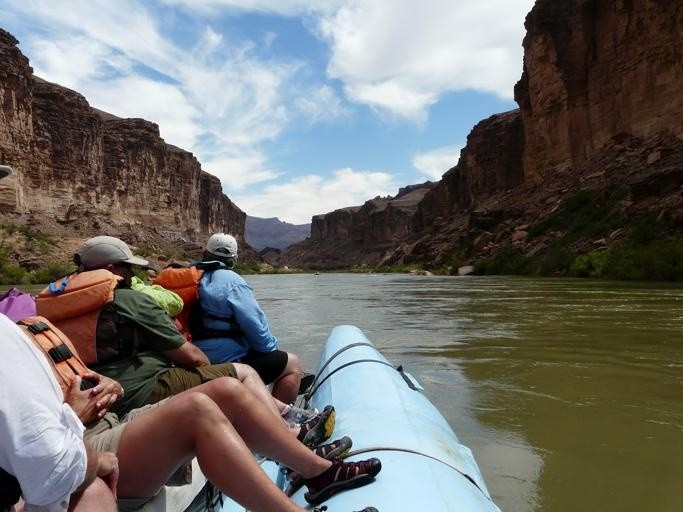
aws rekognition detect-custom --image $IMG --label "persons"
[40,233,351,488]
[0,313,119,512]
[0,288,379,512]
[183,231,314,402]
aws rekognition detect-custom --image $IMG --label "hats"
[207,232,238,258]
[74,236,150,268]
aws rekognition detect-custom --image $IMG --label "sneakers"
[299,374,315,393]
[282,404,320,428]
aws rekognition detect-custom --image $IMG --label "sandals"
[304,458,381,505]
[297,405,336,451]
[280,437,352,488]
